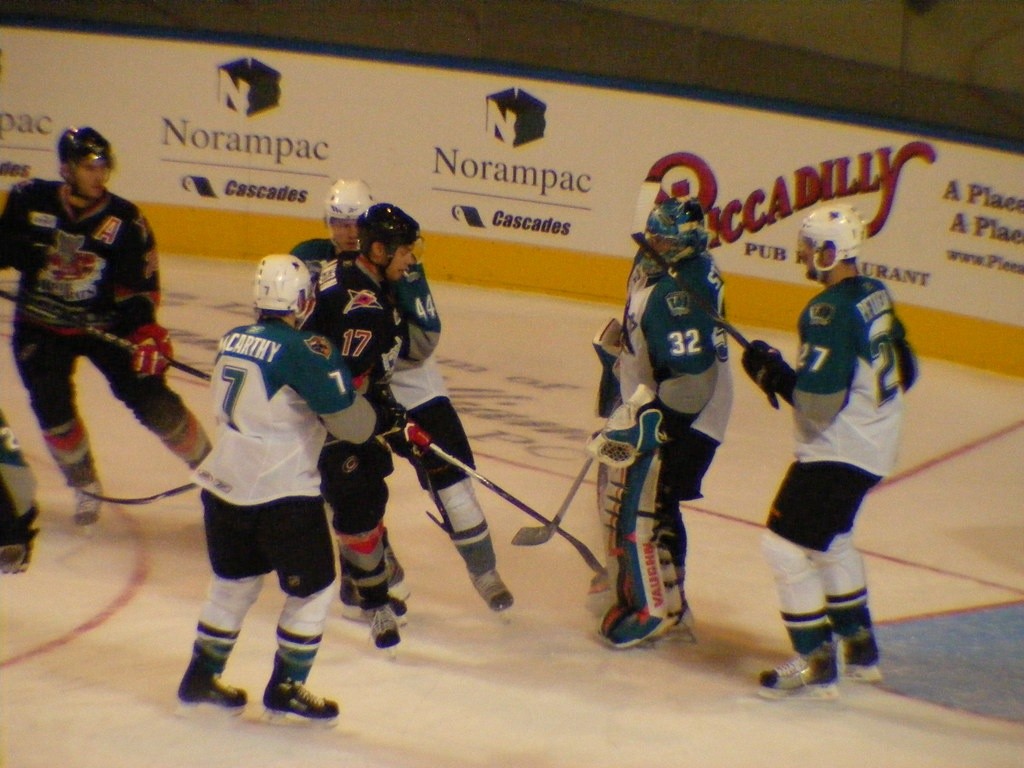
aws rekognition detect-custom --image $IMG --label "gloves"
[132,323,173,377]
[371,402,408,435]
[741,340,796,410]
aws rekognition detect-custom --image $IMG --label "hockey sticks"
[427,443,607,575]
[0,288,210,385]
[631,229,752,348]
[76,437,342,507]
[510,455,593,548]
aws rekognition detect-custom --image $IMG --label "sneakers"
[178,640,247,716]
[758,641,839,700]
[66,469,103,537]
[339,567,407,627]
[466,553,514,623]
[259,651,339,728]
[359,594,401,659]
[836,621,881,683]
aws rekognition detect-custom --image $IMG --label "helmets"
[326,178,372,227]
[356,204,419,245]
[254,254,315,311]
[59,127,113,169]
[798,204,866,271]
[647,198,708,259]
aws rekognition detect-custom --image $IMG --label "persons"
[743,206,918,691]
[593,197,732,649]
[0,128,214,528]
[178,254,377,720]
[286,177,514,649]
